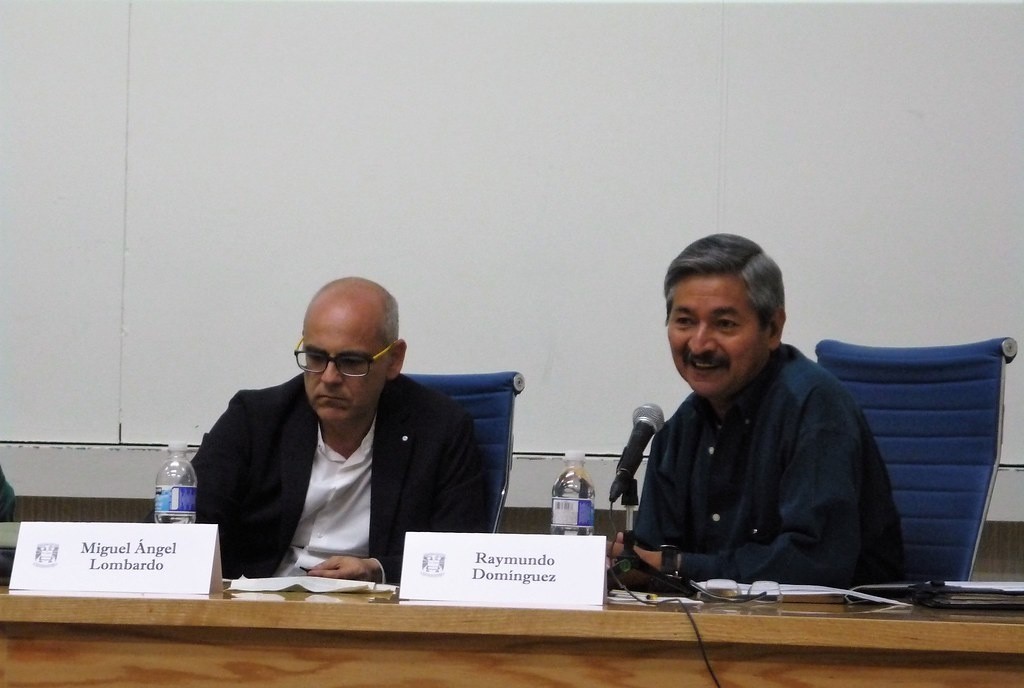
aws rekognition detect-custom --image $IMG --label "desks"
[0,584,1024,687]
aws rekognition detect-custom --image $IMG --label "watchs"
[658,543,683,581]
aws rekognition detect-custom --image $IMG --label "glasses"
[294,337,396,377]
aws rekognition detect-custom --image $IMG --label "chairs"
[407,371,526,533]
[815,336,1020,582]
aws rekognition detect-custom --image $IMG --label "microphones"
[608,402,664,502]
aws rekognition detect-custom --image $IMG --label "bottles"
[548,451,595,536]
[154,443,197,524]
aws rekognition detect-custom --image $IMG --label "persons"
[179,281,490,582]
[592,230,907,595]
